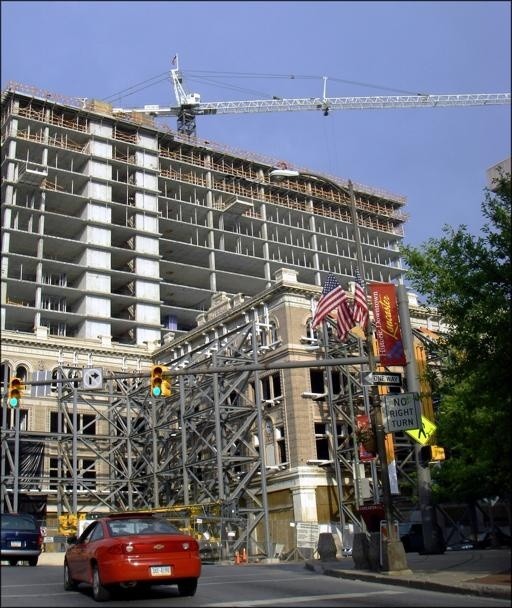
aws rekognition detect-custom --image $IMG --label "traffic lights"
[5,376,24,412]
[149,365,173,401]
[421,446,445,463]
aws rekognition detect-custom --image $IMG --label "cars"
[64,513,202,602]
[0,513,42,566]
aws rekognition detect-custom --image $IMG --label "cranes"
[89,53,510,145]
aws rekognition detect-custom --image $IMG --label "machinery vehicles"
[57,500,224,564]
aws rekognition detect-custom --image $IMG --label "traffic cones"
[234,546,248,565]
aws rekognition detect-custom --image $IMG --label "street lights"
[270,167,409,571]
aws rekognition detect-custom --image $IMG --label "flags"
[311,271,347,333]
[336,300,355,341]
[351,267,370,332]
